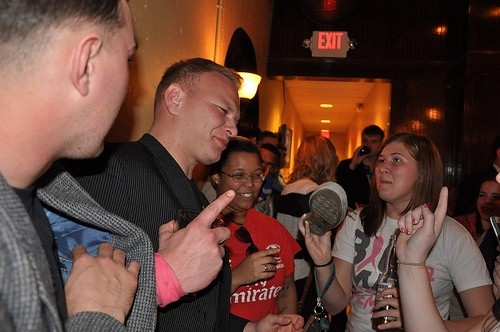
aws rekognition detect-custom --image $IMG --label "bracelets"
[315,257,334,267]
[396,260,426,267]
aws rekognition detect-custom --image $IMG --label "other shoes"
[303,182,347,236]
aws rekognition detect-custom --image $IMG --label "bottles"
[369,235,400,332]
[359,146,372,155]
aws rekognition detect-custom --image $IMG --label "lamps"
[235,72,265,121]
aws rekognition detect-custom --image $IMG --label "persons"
[0,0,157,332]
[200,125,500,332]
[66,57,304,332]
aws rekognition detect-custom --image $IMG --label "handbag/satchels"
[303,312,330,332]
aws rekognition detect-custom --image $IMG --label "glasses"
[216,169,265,184]
[235,227,259,257]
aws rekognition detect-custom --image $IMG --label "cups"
[172,210,222,234]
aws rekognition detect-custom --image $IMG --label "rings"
[264,264,267,271]
